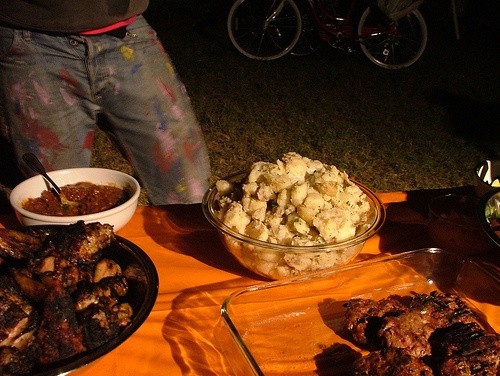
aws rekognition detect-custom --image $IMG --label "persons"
[0,0,212,205]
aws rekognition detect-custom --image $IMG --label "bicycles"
[226,0,429,72]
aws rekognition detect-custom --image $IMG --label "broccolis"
[213,151,371,278]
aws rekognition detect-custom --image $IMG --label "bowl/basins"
[0,225,159,376]
[202,170,386,281]
[212,245,500,375]
[9,167,140,232]
[424,193,492,259]
[476,187,500,247]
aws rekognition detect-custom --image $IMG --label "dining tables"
[0,186,500,376]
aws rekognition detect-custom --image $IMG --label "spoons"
[21,152,79,212]
